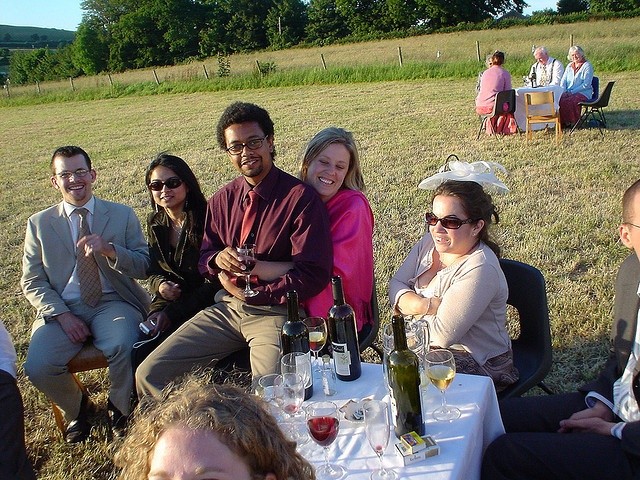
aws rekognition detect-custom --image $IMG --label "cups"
[382,318,430,397]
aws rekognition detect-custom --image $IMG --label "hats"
[417,154,511,191]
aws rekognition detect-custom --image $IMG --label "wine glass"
[305,401,345,480]
[424,350,461,421]
[363,401,398,480]
[303,317,328,379]
[280,352,311,415]
[237,244,259,298]
[256,373,308,446]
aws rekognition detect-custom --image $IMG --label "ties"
[230,190,260,286]
[75,208,102,307]
[540,64,547,84]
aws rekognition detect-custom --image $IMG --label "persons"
[560,45,593,127]
[133,100,334,414]
[1,317,24,479]
[134,154,207,367]
[235,127,375,363]
[388,180,520,395]
[482,179,639,479]
[477,54,493,91]
[524,46,564,88]
[23,147,150,443]
[114,367,318,478]
[475,51,518,133]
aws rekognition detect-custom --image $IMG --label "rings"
[91,244,94,248]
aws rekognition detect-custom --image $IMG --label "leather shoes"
[106,399,126,437]
[66,393,95,443]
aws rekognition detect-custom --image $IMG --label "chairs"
[569,81,615,136]
[53,335,137,437]
[592,77,607,124]
[475,89,523,138]
[497,258,552,398]
[524,92,562,145]
[354,268,389,364]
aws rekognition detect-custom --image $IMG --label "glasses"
[226,135,269,155]
[426,213,472,229]
[148,179,183,191]
[56,168,90,179]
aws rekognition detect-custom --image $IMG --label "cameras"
[138,318,157,336]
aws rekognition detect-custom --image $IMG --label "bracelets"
[425,297,431,315]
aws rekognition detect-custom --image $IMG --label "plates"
[339,397,388,423]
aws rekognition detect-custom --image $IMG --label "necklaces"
[170,224,181,237]
[437,255,456,272]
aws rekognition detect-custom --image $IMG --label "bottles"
[531,66,537,88]
[281,289,313,401]
[322,355,336,396]
[386,315,426,440]
[327,276,362,382]
